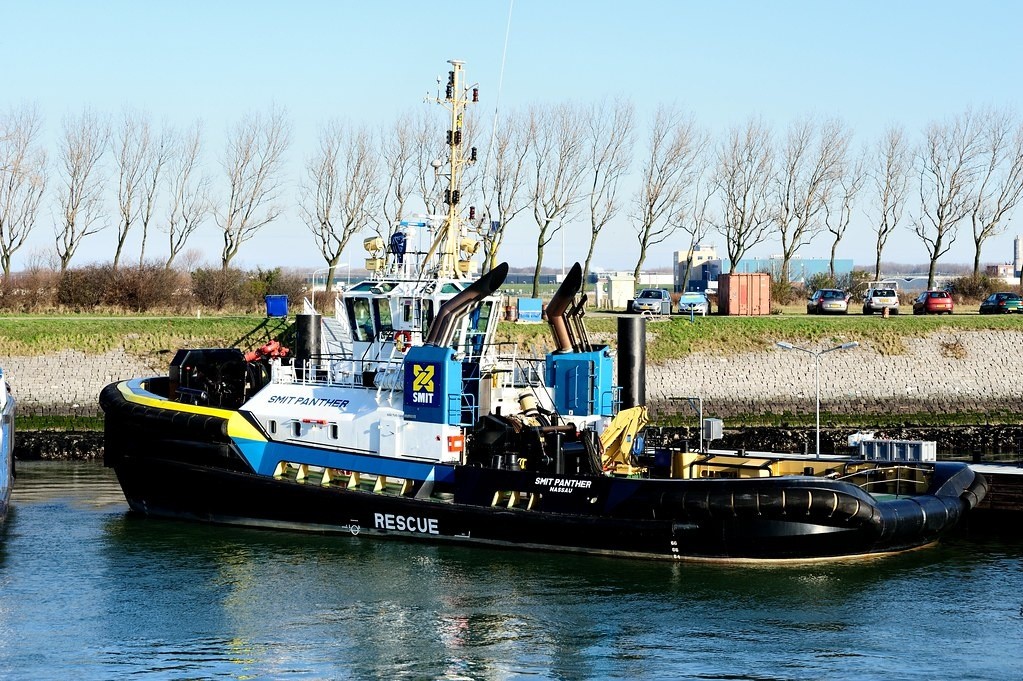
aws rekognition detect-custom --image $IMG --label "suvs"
[862,288,898,315]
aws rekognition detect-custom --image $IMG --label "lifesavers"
[393,330,411,352]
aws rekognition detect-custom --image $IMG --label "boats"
[98,0,990,566]
[0,366,17,528]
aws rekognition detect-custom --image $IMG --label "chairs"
[645,293,653,298]
[885,291,893,296]
[873,291,878,296]
[938,293,945,298]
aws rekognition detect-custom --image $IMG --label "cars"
[807,289,848,316]
[678,292,711,315]
[979,292,1023,314]
[631,288,673,314]
[913,291,953,315]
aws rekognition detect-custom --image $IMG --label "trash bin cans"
[264,294,288,318]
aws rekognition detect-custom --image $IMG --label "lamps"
[428,160,441,169]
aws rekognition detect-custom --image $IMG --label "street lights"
[668,396,703,455]
[311,263,348,309]
[542,217,565,282]
[776,340,861,457]
[705,270,711,281]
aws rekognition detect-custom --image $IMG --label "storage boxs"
[266,294,290,317]
[517,297,542,322]
